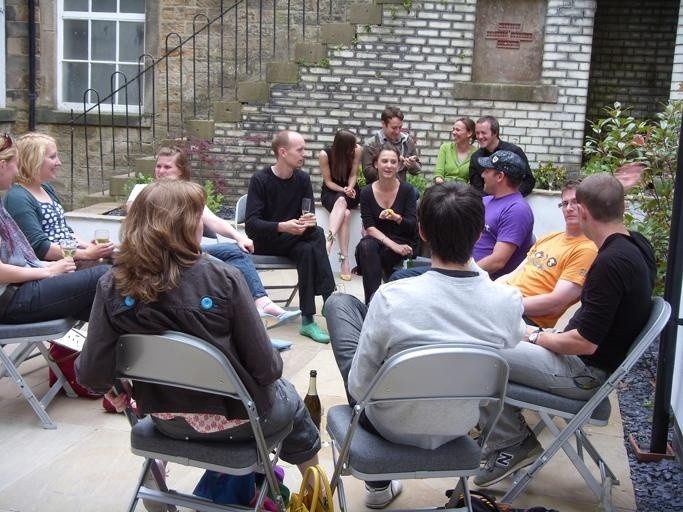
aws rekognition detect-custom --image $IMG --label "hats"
[477,150,527,178]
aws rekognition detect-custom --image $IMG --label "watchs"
[528,329,542,346]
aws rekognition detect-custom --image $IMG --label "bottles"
[304,370,322,425]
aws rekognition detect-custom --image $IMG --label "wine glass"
[301,199,311,217]
[94,231,109,260]
[62,240,76,271]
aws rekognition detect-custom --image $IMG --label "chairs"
[233,194,301,307]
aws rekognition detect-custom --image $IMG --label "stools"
[0,313,79,429]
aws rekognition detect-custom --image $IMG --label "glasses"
[0,133,12,152]
[558,199,577,208]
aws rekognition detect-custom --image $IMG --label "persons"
[243,129,337,344]
[323,177,524,512]
[473,174,660,489]
[71,175,333,511]
[1,131,122,273]
[360,105,424,185]
[432,117,480,186]
[125,142,304,354]
[389,149,536,282]
[497,179,600,332]
[468,115,535,199]
[355,140,425,306]
[1,130,117,325]
[318,127,363,282]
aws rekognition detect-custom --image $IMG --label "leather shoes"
[339,254,351,281]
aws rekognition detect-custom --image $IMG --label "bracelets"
[395,215,402,223]
[381,236,388,244]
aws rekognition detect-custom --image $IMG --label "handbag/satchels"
[50,341,104,400]
[286,493,334,512]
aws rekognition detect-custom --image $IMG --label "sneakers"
[269,338,292,352]
[104,396,137,414]
[365,479,403,509]
[473,433,547,487]
[300,322,330,343]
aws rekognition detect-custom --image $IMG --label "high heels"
[255,301,301,330]
